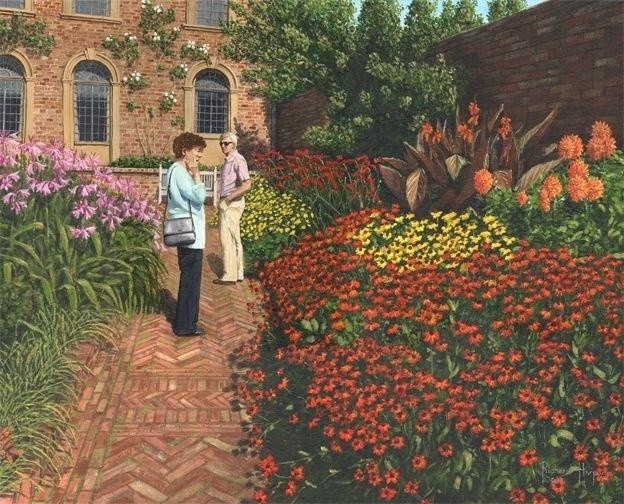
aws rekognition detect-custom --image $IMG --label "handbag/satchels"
[163,217,195,247]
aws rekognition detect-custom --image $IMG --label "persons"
[212,132,252,285]
[164,132,207,336]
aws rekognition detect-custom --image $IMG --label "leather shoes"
[213,279,236,285]
[178,328,203,336]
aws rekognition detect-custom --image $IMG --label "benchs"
[157,162,219,207]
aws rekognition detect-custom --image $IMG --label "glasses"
[220,142,233,146]
